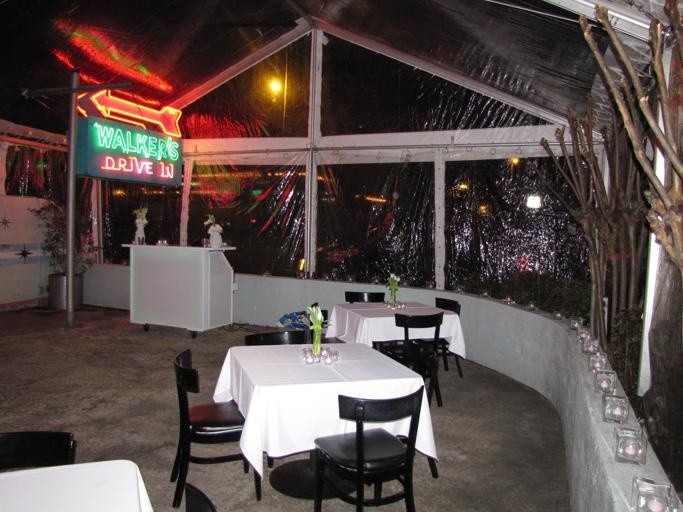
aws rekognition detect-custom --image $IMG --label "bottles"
[131,237,145,245]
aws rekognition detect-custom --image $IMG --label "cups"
[302,347,338,365]
[200,239,210,248]
[382,300,406,310]
[155,240,167,246]
[550,308,673,512]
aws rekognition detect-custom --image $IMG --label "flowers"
[134,205,148,220]
[306,304,330,357]
[203,213,217,230]
[383,271,400,306]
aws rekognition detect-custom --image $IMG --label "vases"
[207,225,223,248]
[134,218,148,246]
